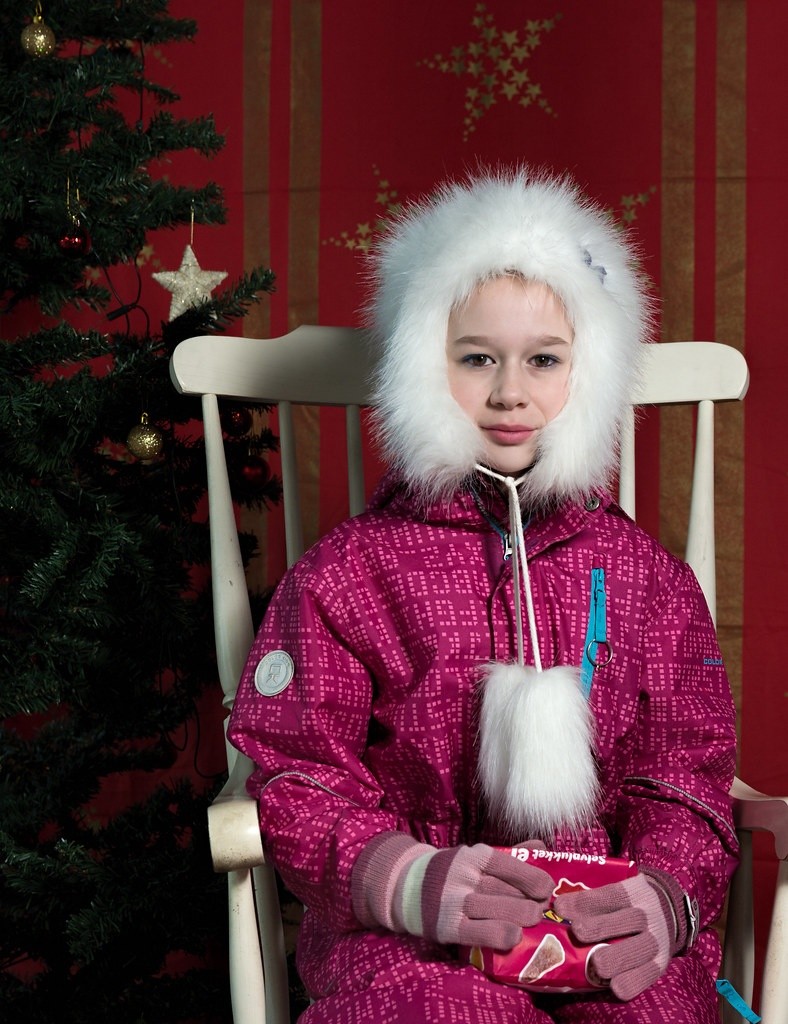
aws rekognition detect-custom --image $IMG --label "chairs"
[171,323,788,1024]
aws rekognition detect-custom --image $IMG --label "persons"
[223,159,737,1024]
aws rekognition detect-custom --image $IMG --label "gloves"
[351,831,556,950]
[550,865,689,1001]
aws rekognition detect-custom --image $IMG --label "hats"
[362,162,656,838]
